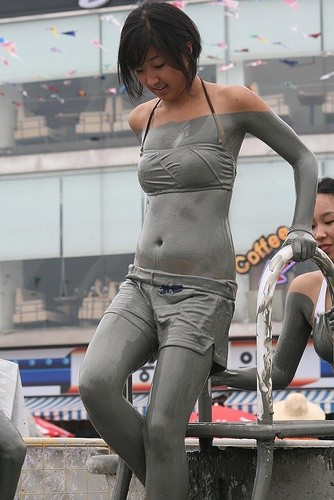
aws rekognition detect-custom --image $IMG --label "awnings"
[223,388,334,421]
[24,392,229,421]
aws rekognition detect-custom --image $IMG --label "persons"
[211,177,334,500]
[0,409,27,500]
[78,0,319,500]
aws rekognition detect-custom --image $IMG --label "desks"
[55,113,79,142]
[299,94,324,126]
[54,297,78,326]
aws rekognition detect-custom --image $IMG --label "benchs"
[78,282,120,327]
[14,103,61,146]
[75,97,134,142]
[12,288,65,329]
[251,82,294,127]
